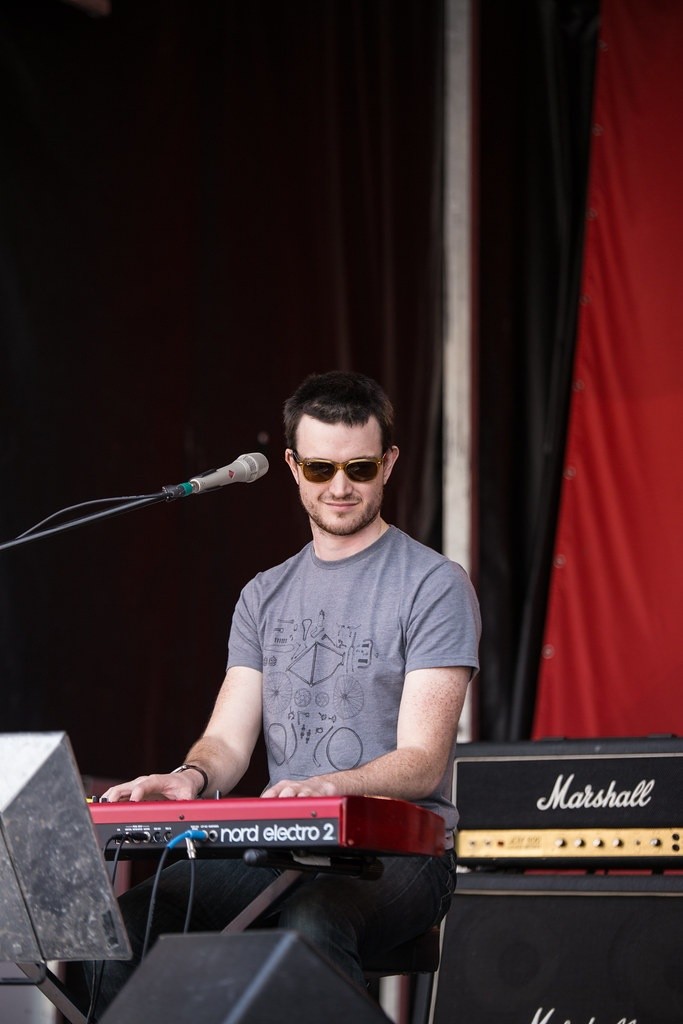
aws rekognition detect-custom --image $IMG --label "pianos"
[89,792,449,858]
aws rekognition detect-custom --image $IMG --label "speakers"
[0,731,683,1024]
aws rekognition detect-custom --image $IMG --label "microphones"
[172,452,269,499]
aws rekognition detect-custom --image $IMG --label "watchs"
[170,764,209,796]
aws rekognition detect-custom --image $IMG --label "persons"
[64,369,482,1022]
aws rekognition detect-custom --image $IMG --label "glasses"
[293,453,387,483]
